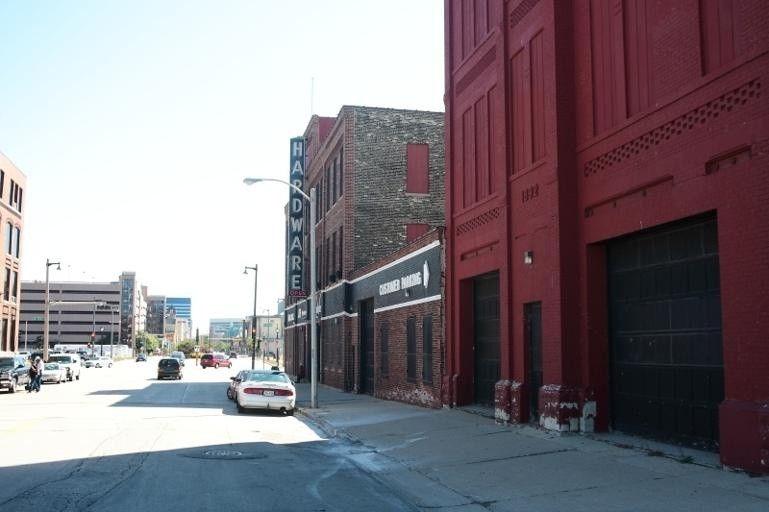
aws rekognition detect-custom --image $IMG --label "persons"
[24,355,44,393]
[293,362,305,383]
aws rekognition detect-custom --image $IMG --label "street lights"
[43,256,61,364]
[242,175,320,410]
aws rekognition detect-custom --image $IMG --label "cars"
[200,353,232,369]
[226,370,297,416]
[0,352,115,394]
[157,358,184,381]
